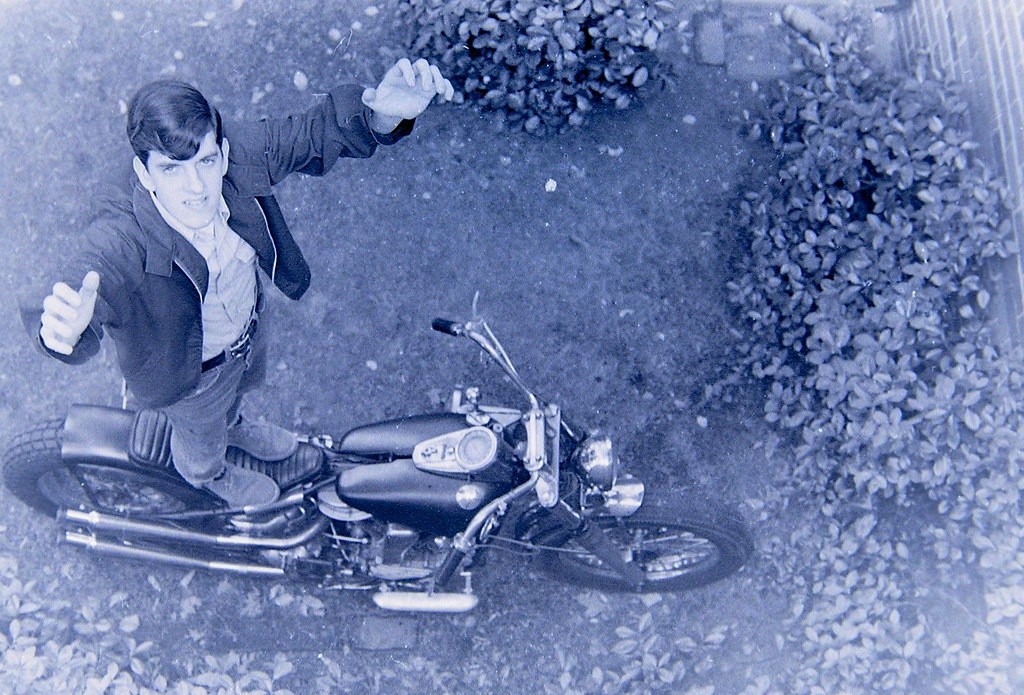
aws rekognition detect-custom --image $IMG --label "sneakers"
[225,413,298,461]
[203,462,279,509]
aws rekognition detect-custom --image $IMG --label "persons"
[15,57,455,512]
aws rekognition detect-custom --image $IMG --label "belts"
[198,310,257,372]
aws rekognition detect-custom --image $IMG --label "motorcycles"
[1,289,755,614]
